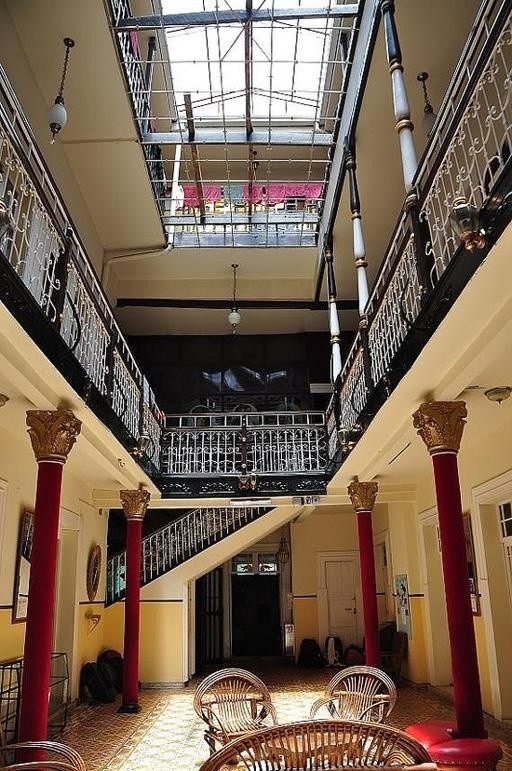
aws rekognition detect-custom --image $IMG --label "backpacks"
[297,635,347,669]
[98,649,141,693]
[82,663,116,702]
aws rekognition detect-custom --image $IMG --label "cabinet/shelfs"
[0,649,68,740]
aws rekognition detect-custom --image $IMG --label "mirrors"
[463,512,482,618]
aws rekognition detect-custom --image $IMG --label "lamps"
[276,535,291,564]
[417,72,437,138]
[47,37,77,144]
[228,263,241,336]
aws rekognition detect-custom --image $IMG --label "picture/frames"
[10,505,38,625]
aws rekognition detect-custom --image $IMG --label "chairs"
[378,631,408,686]
[194,662,437,771]
